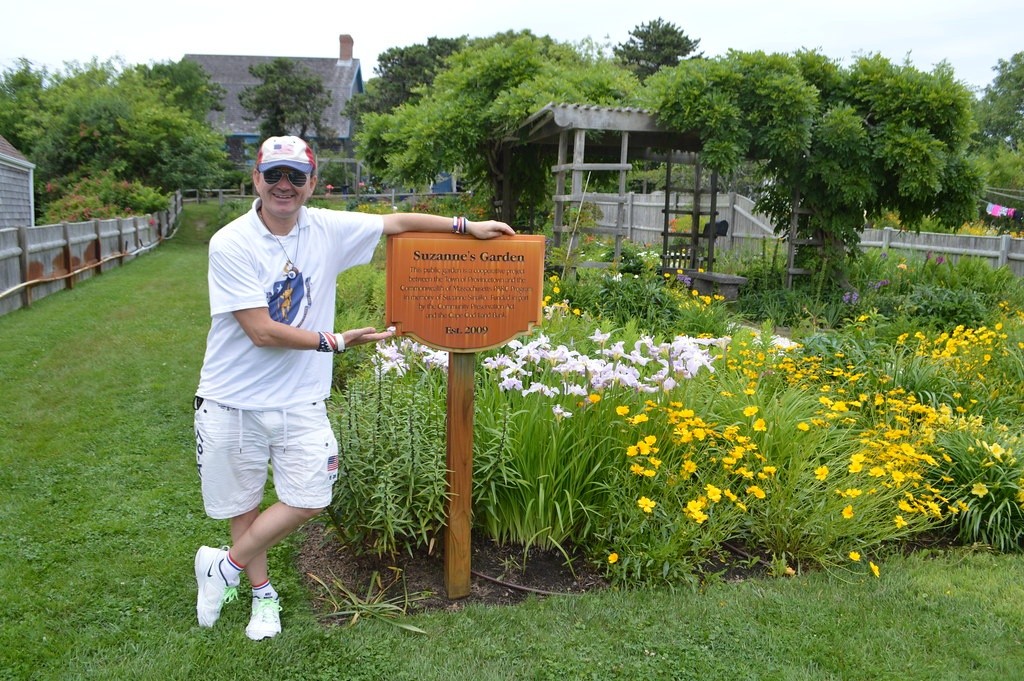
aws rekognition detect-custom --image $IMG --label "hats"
[256,135,317,174]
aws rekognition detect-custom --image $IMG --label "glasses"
[257,169,309,188]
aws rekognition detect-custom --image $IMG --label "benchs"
[687,272,747,302]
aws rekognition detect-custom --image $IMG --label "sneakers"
[245,590,283,642]
[194,544,241,628]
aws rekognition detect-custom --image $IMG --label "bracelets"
[317,330,346,353]
[453,215,466,234]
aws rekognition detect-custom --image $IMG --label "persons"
[194,136,516,640]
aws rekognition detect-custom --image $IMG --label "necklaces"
[262,211,301,279]
